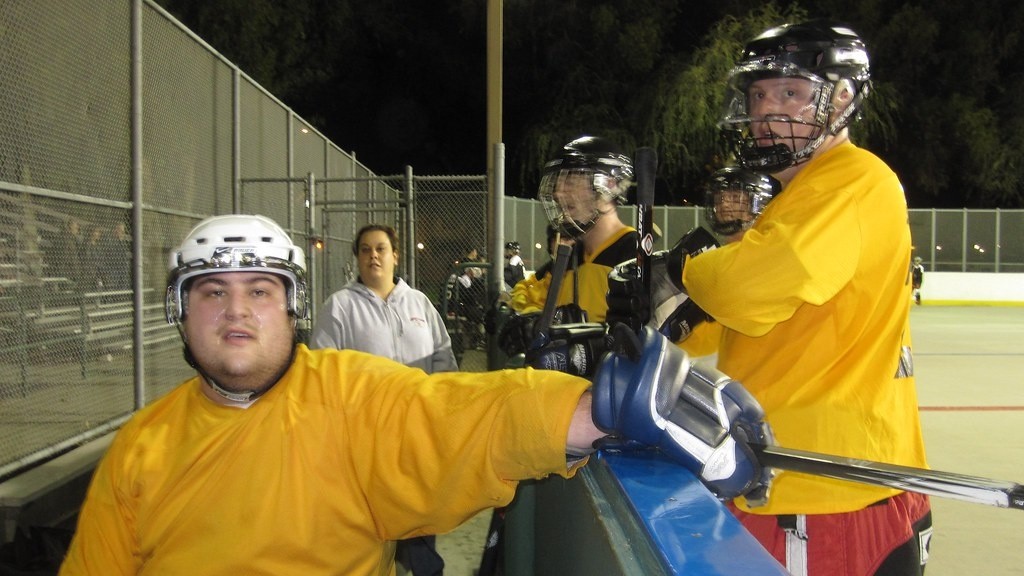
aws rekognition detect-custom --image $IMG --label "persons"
[49,221,127,304]
[446,135,658,375]
[913,257,923,304]
[310,224,458,576]
[606,21,932,576]
[60,214,775,576]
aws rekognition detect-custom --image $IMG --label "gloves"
[606,225,721,362]
[591,322,772,508]
[532,331,607,377]
[499,303,582,358]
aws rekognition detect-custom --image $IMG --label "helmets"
[707,166,776,199]
[737,22,870,94]
[505,242,520,250]
[165,215,306,319]
[541,135,634,173]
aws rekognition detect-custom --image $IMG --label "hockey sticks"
[750,443,1024,514]
[475,241,570,576]
[635,145,659,327]
